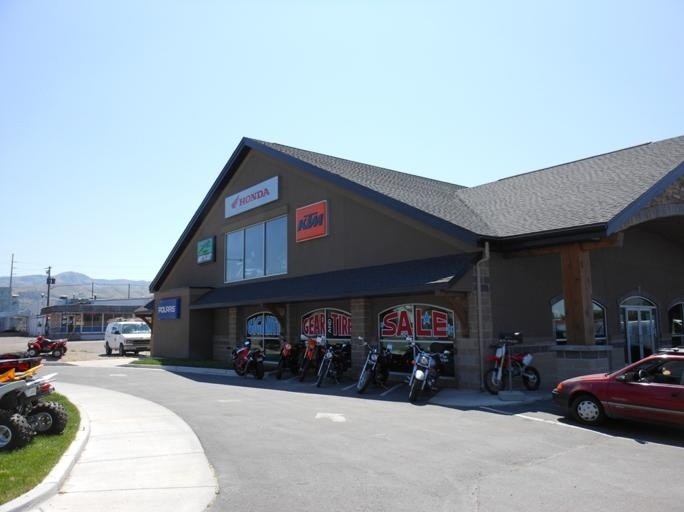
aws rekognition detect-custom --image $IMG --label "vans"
[103,316,152,357]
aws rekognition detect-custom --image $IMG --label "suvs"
[0,349,67,450]
[26,330,70,359]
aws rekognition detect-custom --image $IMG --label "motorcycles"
[479,331,539,396]
[225,321,457,409]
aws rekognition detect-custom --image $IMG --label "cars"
[547,345,683,433]
